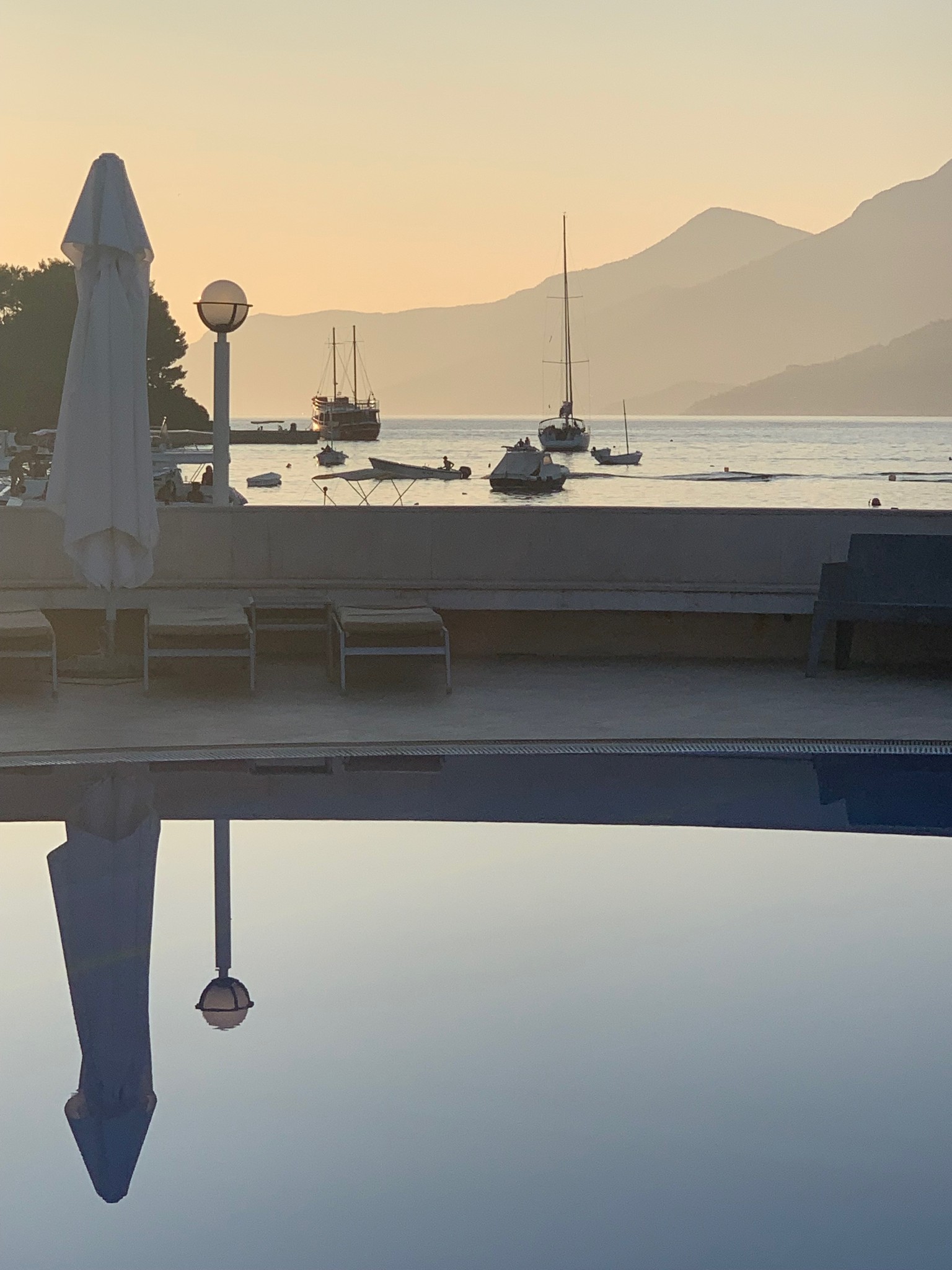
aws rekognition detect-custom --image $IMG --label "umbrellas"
[47,803,161,1203]
[44,151,161,653]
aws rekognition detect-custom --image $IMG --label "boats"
[589,447,611,458]
[246,472,282,487]
[488,446,571,493]
[368,457,472,480]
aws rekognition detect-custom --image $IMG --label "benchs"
[804,533,952,685]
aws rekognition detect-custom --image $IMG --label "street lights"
[192,279,253,504]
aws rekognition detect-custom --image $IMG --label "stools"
[0,609,56,696]
[325,603,454,695]
[143,602,257,690]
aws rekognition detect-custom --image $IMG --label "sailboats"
[314,399,350,466]
[593,399,644,464]
[312,325,382,442]
[537,214,592,453]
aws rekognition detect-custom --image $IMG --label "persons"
[593,446,596,451]
[441,456,454,471]
[525,437,530,445]
[187,482,204,503]
[201,465,213,486]
[517,438,524,447]
[9,445,47,497]
